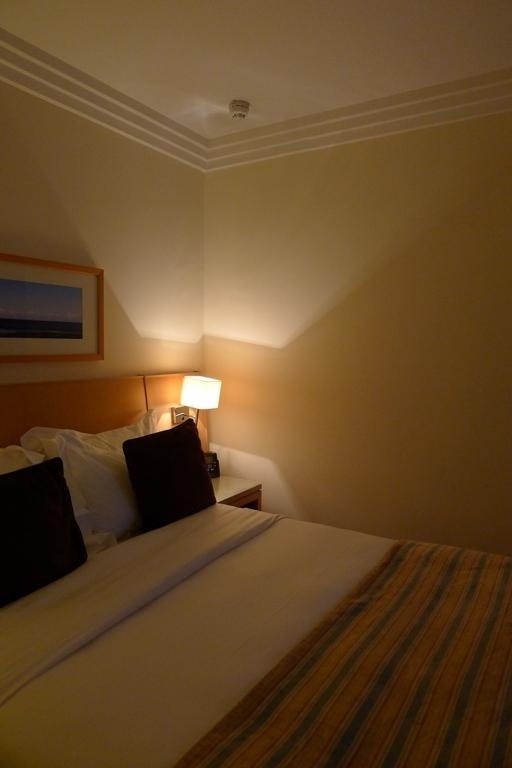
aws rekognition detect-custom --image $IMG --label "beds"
[1,505,512,767]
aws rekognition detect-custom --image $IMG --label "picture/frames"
[0,253,104,362]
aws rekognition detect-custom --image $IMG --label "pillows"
[0,444,41,475]
[1,456,87,607]
[121,418,216,524]
[21,423,147,535]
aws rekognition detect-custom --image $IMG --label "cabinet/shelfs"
[211,477,264,516]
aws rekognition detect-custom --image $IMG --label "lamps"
[180,376,221,427]
